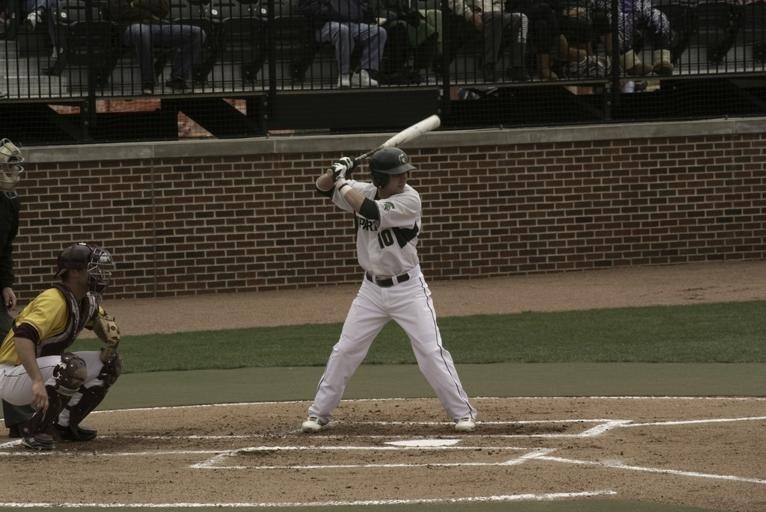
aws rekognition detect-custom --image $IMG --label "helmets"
[58,242,114,295]
[0,138,25,200]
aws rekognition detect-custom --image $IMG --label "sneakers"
[351,69,379,87]
[654,61,674,75]
[337,72,351,87]
[301,415,329,433]
[21,430,57,450]
[453,414,478,432]
[58,425,98,441]
[627,63,653,75]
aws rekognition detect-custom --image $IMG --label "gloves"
[338,156,357,175]
[331,162,347,182]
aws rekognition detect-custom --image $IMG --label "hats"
[368,147,416,175]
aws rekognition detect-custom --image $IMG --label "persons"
[2,1,674,87]
[1,239,125,450]
[298,146,480,437]
[1,132,36,441]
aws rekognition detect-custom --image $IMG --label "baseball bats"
[327,114,441,178]
